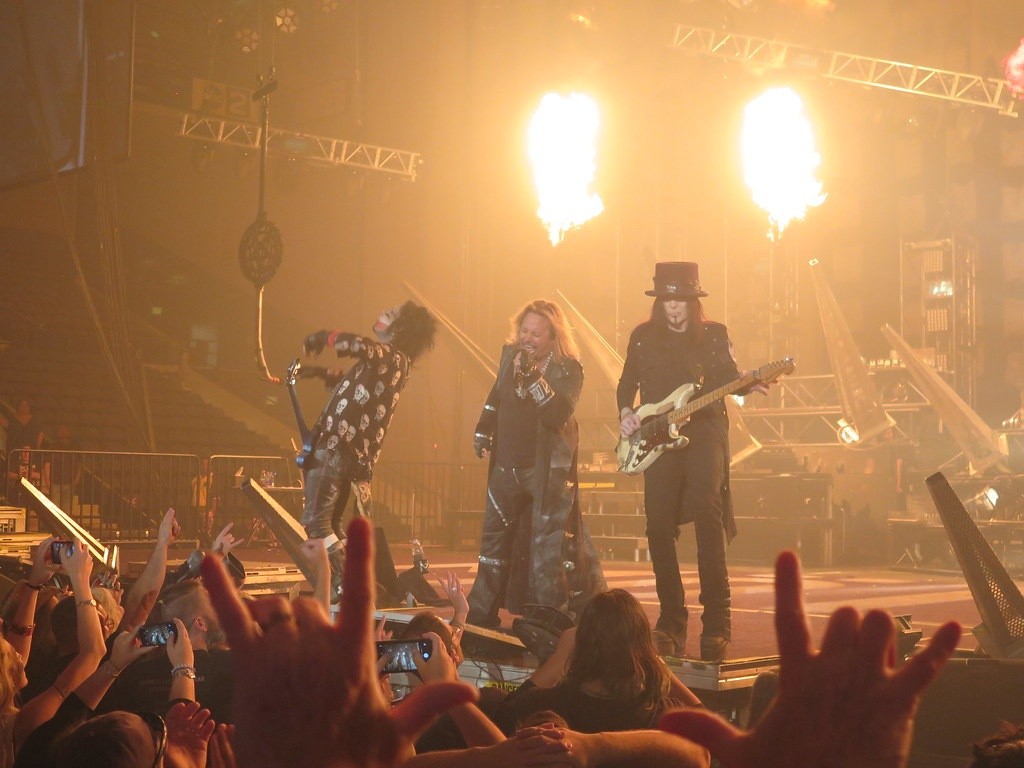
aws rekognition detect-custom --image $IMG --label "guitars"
[616,357,795,476]
[286,358,321,469]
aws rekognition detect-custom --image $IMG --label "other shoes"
[653,620,686,642]
[480,616,502,629]
[699,635,728,652]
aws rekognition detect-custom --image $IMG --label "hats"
[646,261,710,300]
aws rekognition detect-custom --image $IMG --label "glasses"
[450,624,461,648]
[142,713,168,768]
[103,618,114,631]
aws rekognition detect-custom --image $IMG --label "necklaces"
[540,351,553,374]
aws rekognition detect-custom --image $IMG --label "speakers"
[371,526,401,600]
[398,568,450,606]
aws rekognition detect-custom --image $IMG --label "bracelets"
[10,622,35,636]
[26,582,46,590]
[104,662,118,678]
[109,659,120,671]
[171,664,196,679]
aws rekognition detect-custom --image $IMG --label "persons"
[5,398,53,490]
[152,624,174,644]
[467,301,609,627]
[303,301,436,604]
[44,425,85,515]
[0,506,961,768]
[617,261,778,650]
[191,457,218,537]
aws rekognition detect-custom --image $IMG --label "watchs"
[81,598,97,606]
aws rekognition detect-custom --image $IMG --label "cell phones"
[139,622,178,648]
[51,541,76,565]
[375,638,433,673]
